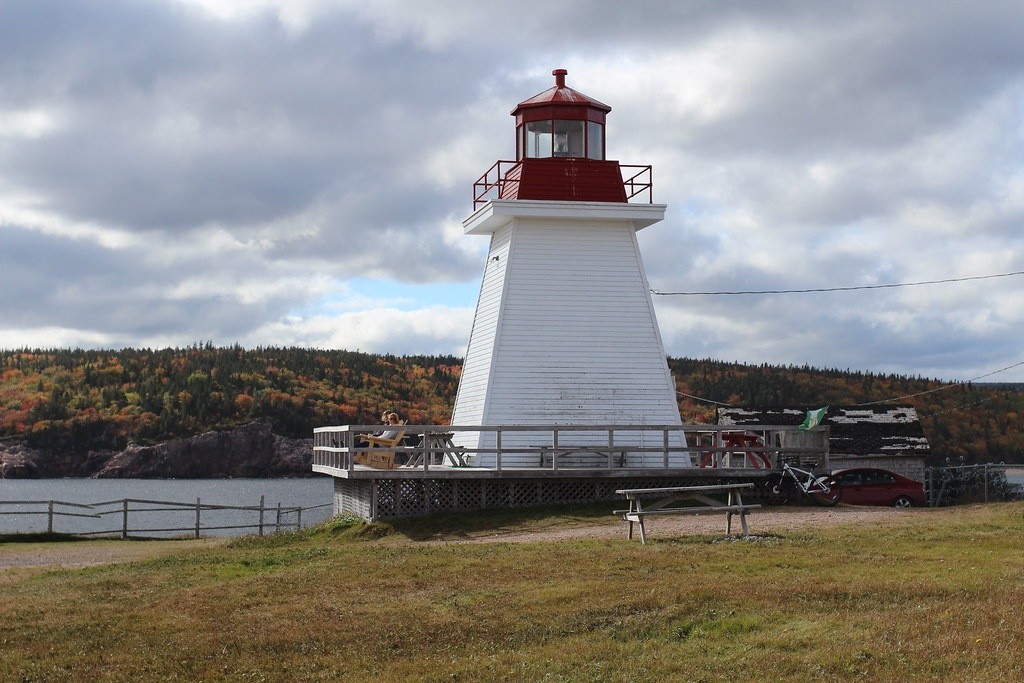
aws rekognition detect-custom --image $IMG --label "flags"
[798,405,830,433]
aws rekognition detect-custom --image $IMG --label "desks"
[406,433,467,468]
[616,483,755,537]
[700,434,771,470]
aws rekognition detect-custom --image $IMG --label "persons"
[330,410,399,453]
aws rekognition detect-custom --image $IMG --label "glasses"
[389,418,393,420]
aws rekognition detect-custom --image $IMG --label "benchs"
[454,445,464,448]
[627,504,762,515]
[404,446,423,449]
[529,445,639,468]
[613,506,713,514]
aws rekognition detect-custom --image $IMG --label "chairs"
[356,420,409,469]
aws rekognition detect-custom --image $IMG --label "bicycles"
[760,457,844,509]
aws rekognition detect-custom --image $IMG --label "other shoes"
[340,441,344,447]
[332,439,339,448]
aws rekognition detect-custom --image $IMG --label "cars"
[801,467,927,508]
